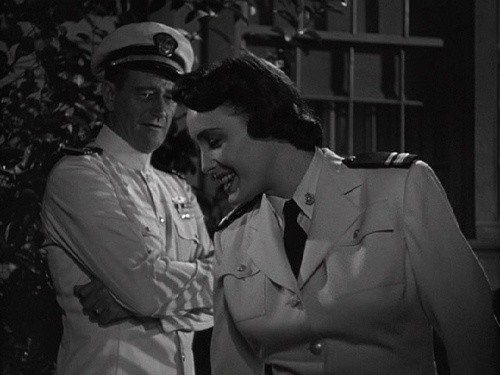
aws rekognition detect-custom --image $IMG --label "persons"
[40,21,215,375]
[174,57,500,374]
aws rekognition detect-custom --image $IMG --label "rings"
[91,306,100,319]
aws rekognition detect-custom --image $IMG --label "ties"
[283,199,308,279]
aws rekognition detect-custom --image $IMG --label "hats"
[91,22,195,82]
